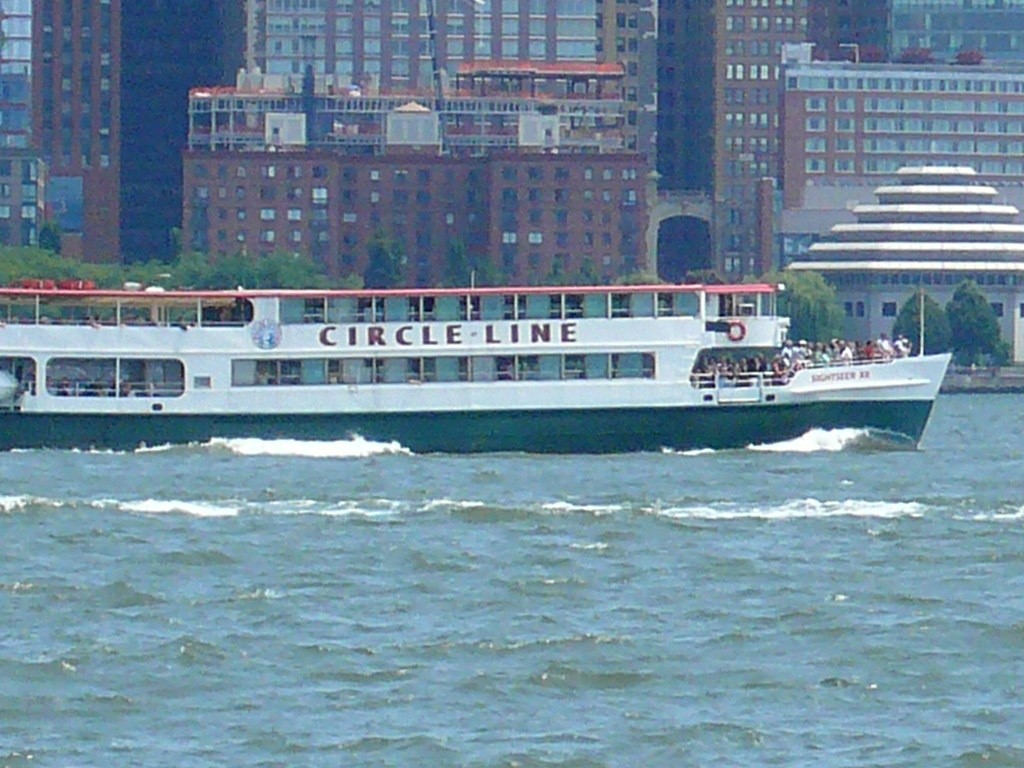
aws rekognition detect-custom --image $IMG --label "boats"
[2,283,952,455]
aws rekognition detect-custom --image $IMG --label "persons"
[0,283,254,330]
[691,333,913,387]
[0,365,185,409]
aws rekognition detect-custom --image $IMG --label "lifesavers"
[727,321,745,341]
